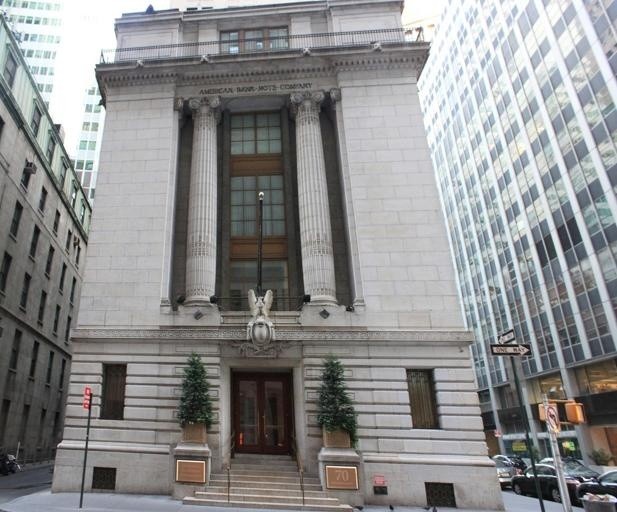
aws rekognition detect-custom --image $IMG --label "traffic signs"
[489,343,531,358]
[497,329,515,344]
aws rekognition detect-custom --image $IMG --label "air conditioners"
[74,237,80,245]
[24,161,37,175]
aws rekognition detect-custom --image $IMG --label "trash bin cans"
[580,498,617,512]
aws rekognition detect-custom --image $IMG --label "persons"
[514,458,527,474]
[512,457,527,474]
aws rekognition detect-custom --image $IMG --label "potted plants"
[177,347,217,443]
[314,351,358,449]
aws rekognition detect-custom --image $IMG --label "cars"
[489,452,617,512]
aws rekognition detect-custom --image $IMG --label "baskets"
[180,420,208,444]
[323,420,351,448]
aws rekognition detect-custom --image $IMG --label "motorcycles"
[0,454,20,476]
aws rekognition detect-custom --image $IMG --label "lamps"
[318,304,329,319]
[176,295,185,303]
[210,295,217,304]
[303,295,310,304]
[193,304,203,320]
[346,305,355,312]
